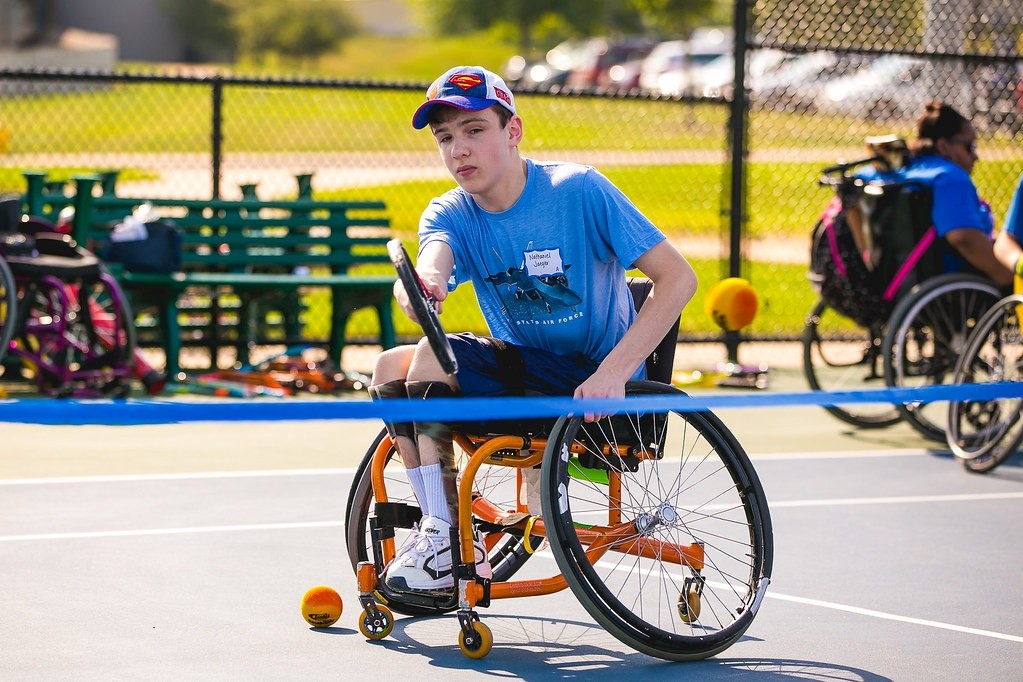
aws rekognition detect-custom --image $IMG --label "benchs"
[7,167,401,380]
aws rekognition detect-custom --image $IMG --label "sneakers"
[384,514,493,592]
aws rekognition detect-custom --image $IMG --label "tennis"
[703,275,760,334]
[302,582,345,628]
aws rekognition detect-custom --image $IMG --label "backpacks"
[811,182,893,326]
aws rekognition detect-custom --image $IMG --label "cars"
[517,27,978,128]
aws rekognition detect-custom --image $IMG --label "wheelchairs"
[950,258,1023,476]
[803,134,1019,450]
[343,271,771,666]
[0,199,142,405]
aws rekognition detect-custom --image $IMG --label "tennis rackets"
[385,235,462,379]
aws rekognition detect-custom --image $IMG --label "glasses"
[943,136,976,153]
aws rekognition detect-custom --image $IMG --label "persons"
[369,65,698,597]
[993,172,1023,276]
[841,108,1016,299]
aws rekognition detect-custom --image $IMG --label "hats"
[412,66,516,130]
